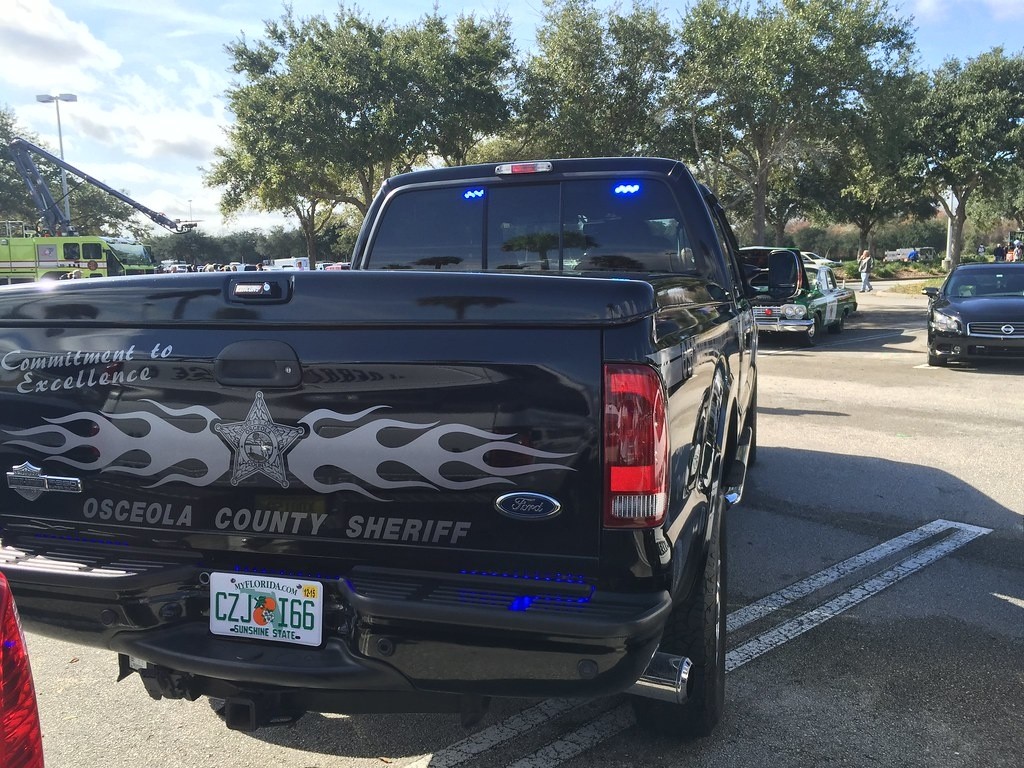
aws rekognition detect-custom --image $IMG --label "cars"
[156,257,349,272]
[739,247,858,347]
[921,262,1024,366]
[800,252,843,267]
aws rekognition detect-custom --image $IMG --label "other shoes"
[867,288,873,292]
[859,290,865,293]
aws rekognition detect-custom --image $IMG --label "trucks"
[884,247,936,262]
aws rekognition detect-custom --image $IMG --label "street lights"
[37,93,77,220]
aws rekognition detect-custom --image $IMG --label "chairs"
[1006,275,1024,291]
[951,276,978,296]
[577,218,679,271]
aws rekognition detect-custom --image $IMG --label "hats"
[590,216,673,251]
[72,269,84,278]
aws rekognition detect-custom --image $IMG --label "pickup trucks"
[0,155,758,740]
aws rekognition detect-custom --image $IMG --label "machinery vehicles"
[0,137,198,283]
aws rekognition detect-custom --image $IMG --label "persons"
[297,261,304,271]
[171,261,263,274]
[908,247,918,261]
[71,270,83,279]
[859,249,872,292]
[977,244,985,256]
[994,237,1024,261]
